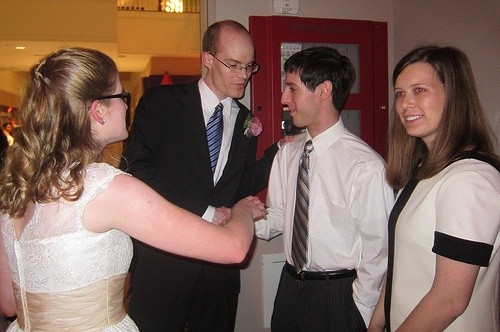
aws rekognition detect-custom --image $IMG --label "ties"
[290,140,313,275]
[206,102,224,175]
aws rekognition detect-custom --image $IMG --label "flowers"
[243,113,263,138]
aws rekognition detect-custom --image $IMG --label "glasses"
[209,52,259,73]
[93,89,130,105]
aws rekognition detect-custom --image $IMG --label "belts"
[284,261,356,281]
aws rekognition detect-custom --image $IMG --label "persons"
[118,20,296,332]
[216,46,393,332]
[0,47,269,332]
[367,46,500,332]
[0,122,14,167]
